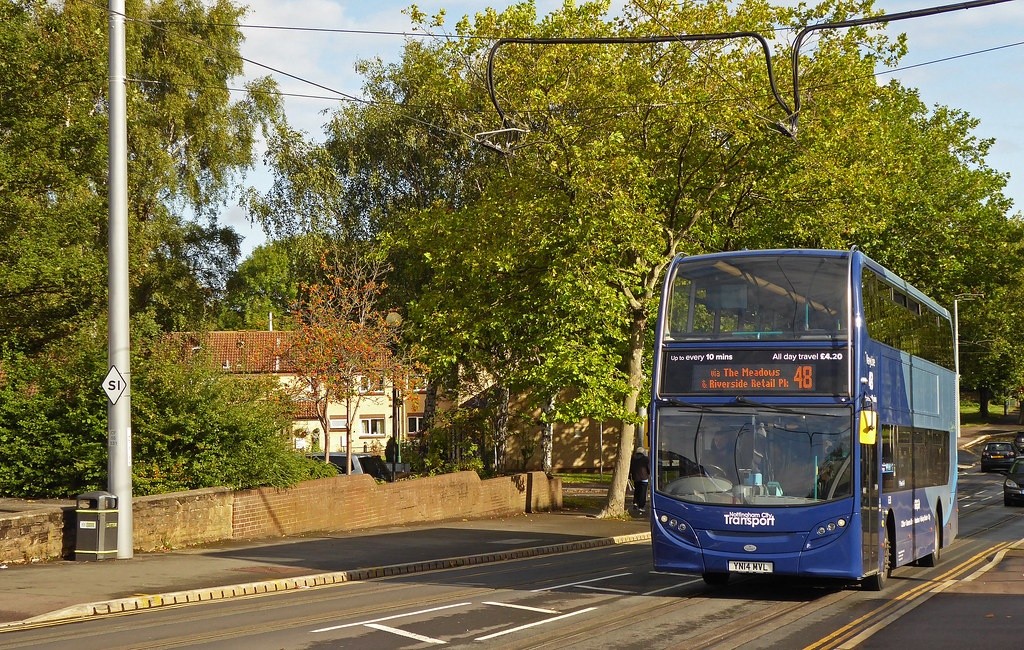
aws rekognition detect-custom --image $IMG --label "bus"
[646,244,960,592]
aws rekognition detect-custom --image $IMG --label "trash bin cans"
[74,490,119,562]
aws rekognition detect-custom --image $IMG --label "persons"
[701,431,741,478]
[629,446,650,513]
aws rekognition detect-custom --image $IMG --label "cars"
[304,451,392,484]
[999,455,1024,507]
[981,441,1021,473]
[1013,431,1024,453]
